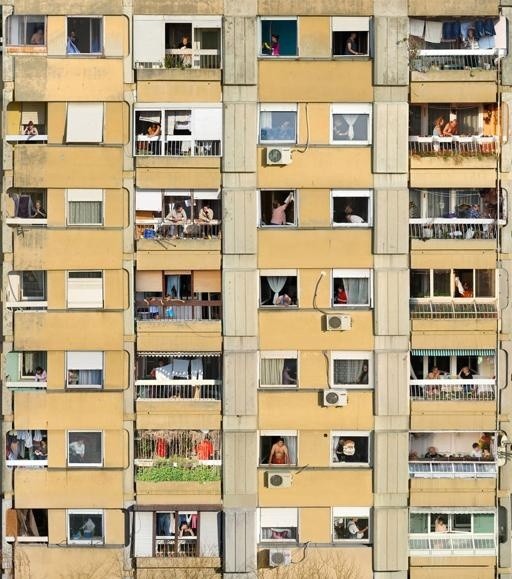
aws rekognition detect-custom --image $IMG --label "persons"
[467,203,482,219]
[440,119,459,150]
[359,364,367,384]
[71,370,79,384]
[24,120,39,144]
[282,364,297,385]
[30,28,44,45]
[433,515,446,549]
[176,35,191,69]
[198,206,213,238]
[425,447,441,465]
[270,192,293,225]
[165,204,188,240]
[345,214,366,223]
[457,366,474,400]
[150,359,164,377]
[344,32,363,55]
[454,279,472,298]
[11,437,21,460]
[164,294,185,319]
[31,200,47,225]
[336,286,347,304]
[70,437,86,463]
[264,34,280,56]
[70,29,79,47]
[144,297,163,319]
[461,27,479,67]
[195,434,214,460]
[482,448,489,457]
[269,438,290,467]
[470,443,482,457]
[347,518,368,539]
[147,123,161,155]
[427,366,440,397]
[333,118,349,140]
[272,531,288,539]
[35,439,48,460]
[276,293,292,308]
[432,116,445,152]
[179,521,195,557]
[35,367,47,389]
[336,439,360,462]
[78,515,96,538]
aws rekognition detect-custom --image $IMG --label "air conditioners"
[267,472,291,488]
[268,549,292,567]
[326,313,351,330]
[322,388,348,407]
[266,146,290,165]
